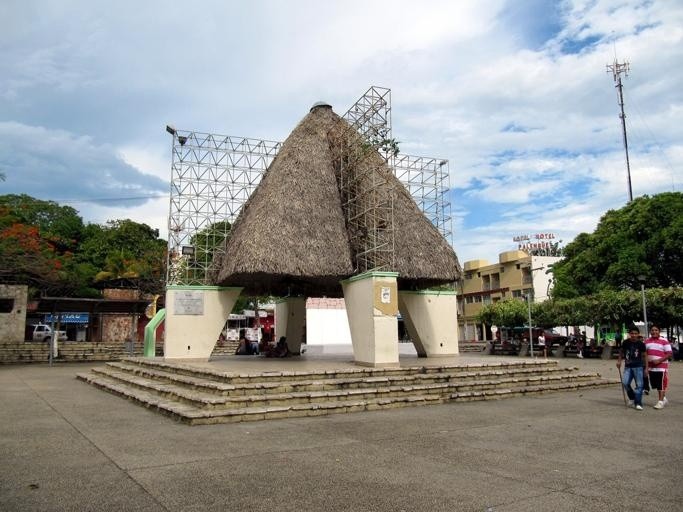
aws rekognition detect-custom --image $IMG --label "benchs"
[492,342,620,358]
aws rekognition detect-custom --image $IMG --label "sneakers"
[628,389,668,411]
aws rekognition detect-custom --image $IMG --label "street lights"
[522,293,533,358]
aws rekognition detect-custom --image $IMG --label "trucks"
[594,322,647,360]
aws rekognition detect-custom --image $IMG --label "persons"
[480,322,681,411]
[218,319,289,359]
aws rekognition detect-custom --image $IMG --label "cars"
[26,322,68,344]
[527,328,571,347]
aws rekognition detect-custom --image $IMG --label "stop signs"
[490,325,497,332]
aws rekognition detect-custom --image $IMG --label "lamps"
[182,246,194,255]
[178,136,187,146]
[166,125,174,136]
